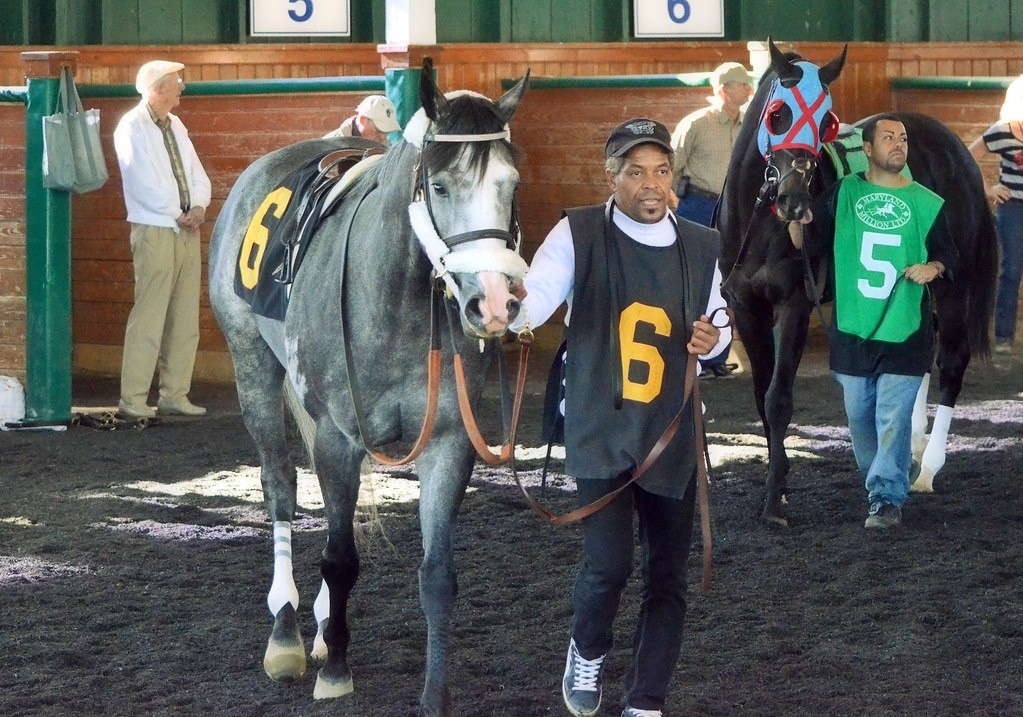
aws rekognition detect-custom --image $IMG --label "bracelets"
[926,262,942,275]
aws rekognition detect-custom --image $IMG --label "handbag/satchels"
[42,64,109,194]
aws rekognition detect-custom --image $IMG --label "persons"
[114,59,212,420]
[321,95,402,147]
[789,112,958,527]
[967,74,1023,355]
[509,119,733,717]
[670,61,759,228]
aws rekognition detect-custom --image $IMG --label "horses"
[717,34,998,528]
[208,55,530,717]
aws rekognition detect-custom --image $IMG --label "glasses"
[167,77,183,87]
[369,121,389,135]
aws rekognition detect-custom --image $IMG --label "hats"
[604,118,674,158]
[358,96,402,132]
[135,61,185,93]
[711,62,756,85]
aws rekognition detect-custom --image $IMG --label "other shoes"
[118,400,156,418]
[733,330,740,340]
[712,363,730,376]
[996,345,1012,355]
[697,364,716,379]
[157,397,207,415]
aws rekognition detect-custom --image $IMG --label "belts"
[687,186,719,200]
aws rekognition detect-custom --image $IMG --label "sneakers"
[561,637,606,717]
[864,493,902,527]
[622,706,662,717]
